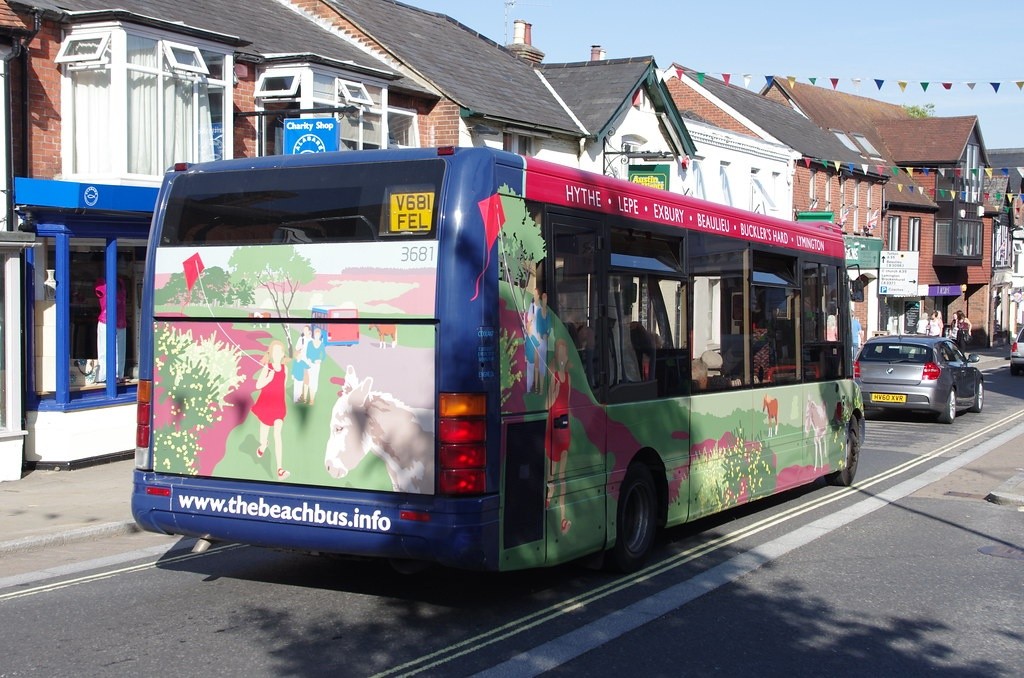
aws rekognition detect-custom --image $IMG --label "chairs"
[901,354,908,358]
[884,348,901,358]
[914,354,926,359]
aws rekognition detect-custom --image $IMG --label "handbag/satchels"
[963,329,976,344]
[926,321,930,336]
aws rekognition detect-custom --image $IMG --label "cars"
[1008,323,1024,375]
[853,333,985,424]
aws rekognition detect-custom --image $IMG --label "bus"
[128,144,868,578]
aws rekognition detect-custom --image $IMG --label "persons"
[555,297,662,385]
[752,291,776,384]
[692,349,733,393]
[916,309,973,358]
[826,305,863,364]
[95,271,127,382]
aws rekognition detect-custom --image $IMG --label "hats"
[700,351,723,377]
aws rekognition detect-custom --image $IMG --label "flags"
[867,207,879,236]
[808,197,820,211]
[835,206,849,228]
